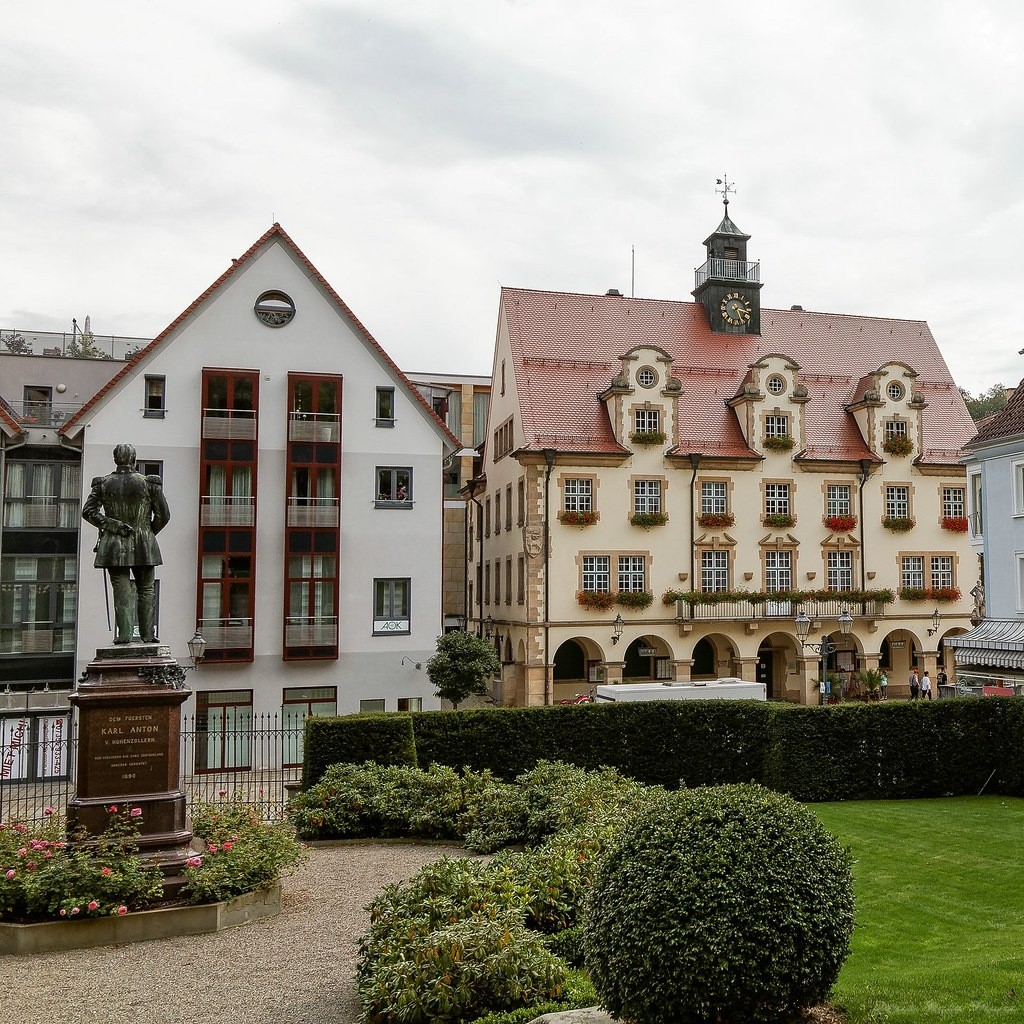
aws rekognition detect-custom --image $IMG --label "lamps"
[181,630,208,675]
[484,613,503,642]
[611,613,625,645]
[927,608,941,637]
[457,613,475,636]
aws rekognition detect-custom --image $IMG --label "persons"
[838,668,858,692]
[879,670,888,700]
[909,669,933,700]
[81,443,171,644]
[936,667,948,699]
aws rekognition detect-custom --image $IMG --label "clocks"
[721,292,752,326]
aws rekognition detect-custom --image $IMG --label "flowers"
[760,513,797,530]
[881,515,916,535]
[577,587,656,615]
[660,586,895,611]
[881,432,914,458]
[630,431,669,451]
[900,587,963,604]
[761,434,797,455]
[698,509,736,531]
[823,513,858,533]
[630,512,669,533]
[941,517,968,534]
[559,510,601,530]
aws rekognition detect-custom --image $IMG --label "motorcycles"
[559,688,594,705]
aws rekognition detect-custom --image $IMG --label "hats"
[914,669,919,673]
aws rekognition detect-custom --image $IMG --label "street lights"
[795,608,854,706]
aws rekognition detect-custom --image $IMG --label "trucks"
[595,678,767,704]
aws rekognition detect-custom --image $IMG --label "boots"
[884,695,887,700]
[880,695,884,701]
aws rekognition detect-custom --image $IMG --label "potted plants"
[856,665,894,701]
[810,671,841,705]
[19,410,40,425]
